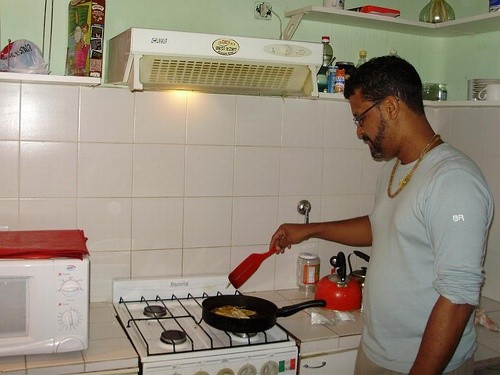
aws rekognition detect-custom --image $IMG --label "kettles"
[314,252,362,311]
[348,250,370,288]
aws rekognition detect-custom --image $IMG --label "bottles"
[419,0,455,24]
[334,62,354,94]
[355,50,367,68]
[438,83,447,102]
[321,35,334,67]
[296,253,321,292]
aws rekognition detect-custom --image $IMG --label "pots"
[201,294,326,333]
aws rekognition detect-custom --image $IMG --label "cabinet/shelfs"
[282,5,500,107]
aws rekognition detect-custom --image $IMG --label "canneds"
[326,61,357,94]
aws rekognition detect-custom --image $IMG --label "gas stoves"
[112,277,298,375]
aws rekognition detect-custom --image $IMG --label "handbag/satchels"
[0,39,52,75]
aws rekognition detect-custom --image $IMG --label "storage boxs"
[65,0,106,79]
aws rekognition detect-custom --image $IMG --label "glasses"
[351,95,399,127]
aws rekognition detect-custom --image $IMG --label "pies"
[211,305,256,319]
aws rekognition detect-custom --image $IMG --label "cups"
[323,0,345,10]
[477,84,500,101]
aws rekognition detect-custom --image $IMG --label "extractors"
[106,27,325,100]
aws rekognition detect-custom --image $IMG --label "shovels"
[228,247,280,290]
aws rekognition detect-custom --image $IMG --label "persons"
[270,55,493,375]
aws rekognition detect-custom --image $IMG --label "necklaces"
[387,134,440,198]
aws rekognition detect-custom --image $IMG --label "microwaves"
[0,255,90,357]
